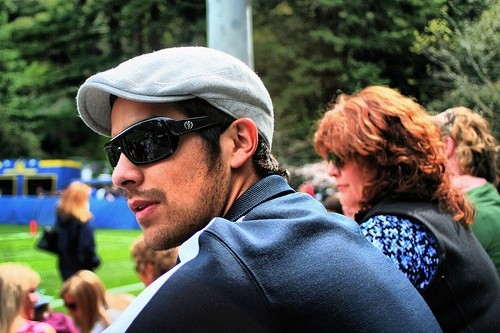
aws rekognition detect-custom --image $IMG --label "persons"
[312,84,500,333]
[76,46,442,333]
[56,181,100,283]
[0,238,178,333]
[287,106,500,277]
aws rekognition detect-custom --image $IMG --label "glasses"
[102,113,228,166]
[64,303,77,310]
[325,150,347,168]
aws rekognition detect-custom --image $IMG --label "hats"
[75,46,274,153]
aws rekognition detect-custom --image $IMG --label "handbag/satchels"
[37,226,59,255]
